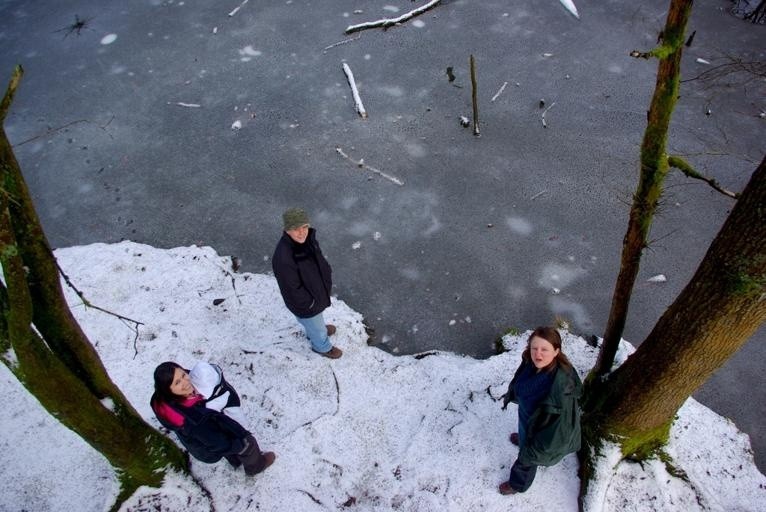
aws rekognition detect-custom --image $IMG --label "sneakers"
[250,452,275,475]
[510,433,519,445]
[312,346,342,359]
[499,482,519,495]
[305,324,336,340]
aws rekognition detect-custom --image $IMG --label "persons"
[272,207,343,359]
[498,325,586,495]
[147,360,277,476]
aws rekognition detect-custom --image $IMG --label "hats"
[283,208,310,231]
